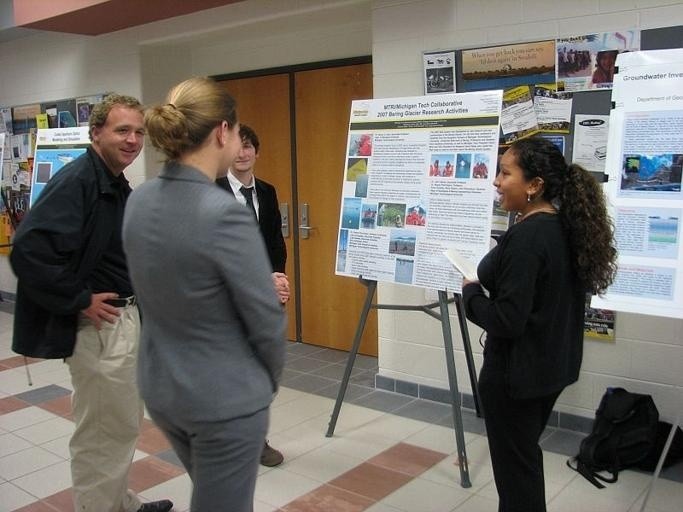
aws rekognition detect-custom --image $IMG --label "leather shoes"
[137,499,173,512]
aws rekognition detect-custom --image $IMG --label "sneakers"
[261,442,283,466]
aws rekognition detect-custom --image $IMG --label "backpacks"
[565,386,664,490]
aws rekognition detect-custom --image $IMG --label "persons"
[558,48,591,78]
[430,160,440,176]
[473,161,488,179]
[373,211,377,227]
[378,204,386,226]
[593,50,618,83]
[216,123,291,467]
[443,161,453,176]
[364,208,372,226]
[121,76,289,512]
[406,206,425,226]
[462,136,617,512]
[8,92,173,512]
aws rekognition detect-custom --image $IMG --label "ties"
[239,187,258,220]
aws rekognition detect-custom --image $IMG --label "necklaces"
[522,209,556,220]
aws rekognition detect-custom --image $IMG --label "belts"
[102,294,137,309]
